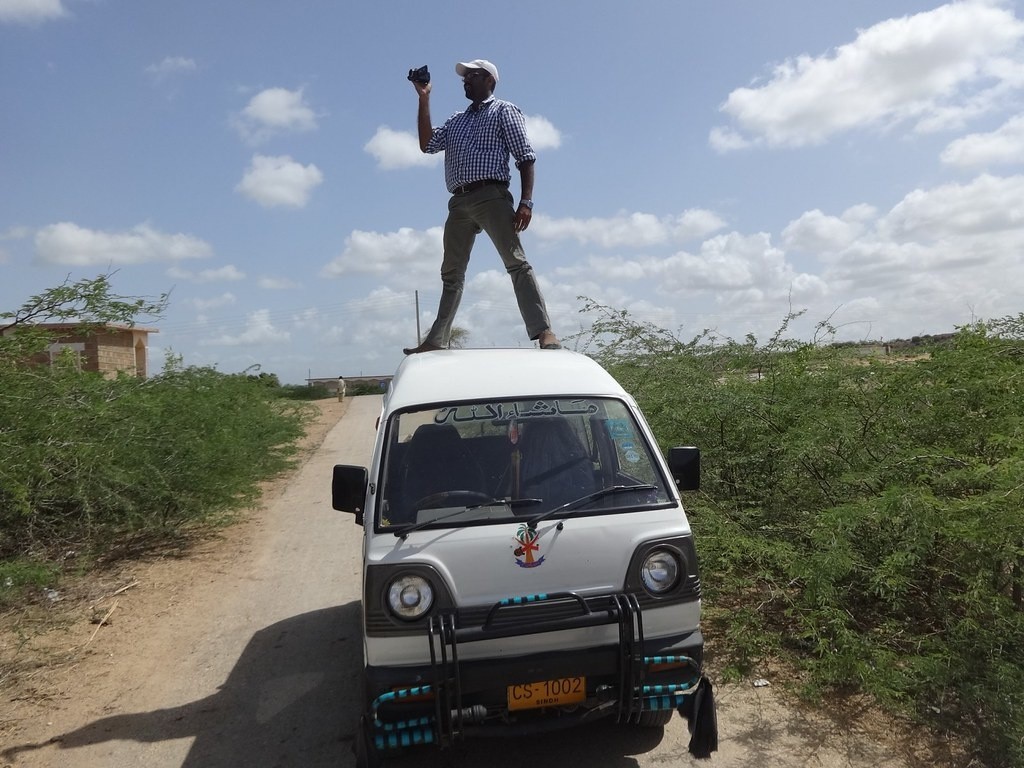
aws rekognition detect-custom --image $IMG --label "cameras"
[407,65,431,85]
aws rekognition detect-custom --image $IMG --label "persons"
[335,376,346,401]
[403,59,562,355]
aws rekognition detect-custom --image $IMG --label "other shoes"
[403,340,446,355]
[539,331,561,349]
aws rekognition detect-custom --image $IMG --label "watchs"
[520,198,534,209]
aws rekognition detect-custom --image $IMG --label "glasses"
[462,71,489,81]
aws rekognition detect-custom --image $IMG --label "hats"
[455,59,500,84]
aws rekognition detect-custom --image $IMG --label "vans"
[327,348,707,768]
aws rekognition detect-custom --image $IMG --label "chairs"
[517,416,599,511]
[402,424,489,520]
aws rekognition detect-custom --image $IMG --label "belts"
[455,180,508,193]
